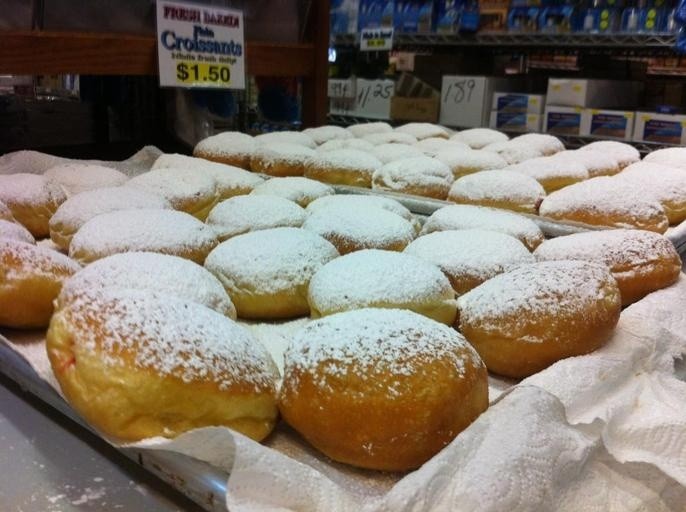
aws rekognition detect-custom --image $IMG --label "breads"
[194,122,685,236]
[0,154,682,473]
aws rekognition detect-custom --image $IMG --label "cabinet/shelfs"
[327,29,684,159]
